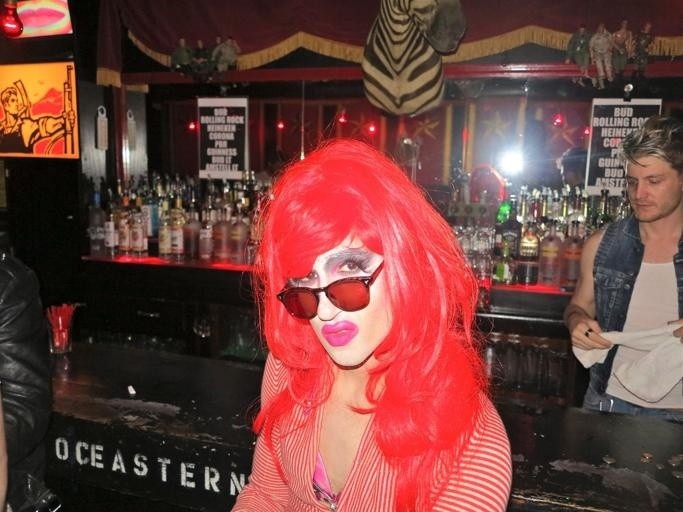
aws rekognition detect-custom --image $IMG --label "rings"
[584,328,593,338]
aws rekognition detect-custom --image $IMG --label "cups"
[194,317,215,344]
[48,315,72,353]
[229,314,259,361]
[137,300,171,348]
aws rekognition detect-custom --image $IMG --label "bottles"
[198,207,213,261]
[118,189,131,254]
[591,188,610,234]
[103,187,118,256]
[182,205,201,257]
[562,220,583,281]
[615,190,630,223]
[232,208,250,263]
[87,185,106,257]
[171,209,186,256]
[157,200,172,255]
[213,208,232,262]
[518,181,590,239]
[500,200,523,259]
[496,241,513,283]
[154,169,262,220]
[539,219,561,281]
[517,222,540,285]
[131,192,147,254]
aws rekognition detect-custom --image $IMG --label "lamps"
[0,1,23,40]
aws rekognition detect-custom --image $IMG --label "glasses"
[277,255,385,321]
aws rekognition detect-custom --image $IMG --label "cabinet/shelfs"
[81,221,576,406]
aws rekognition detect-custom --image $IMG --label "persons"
[1,244,53,511]
[0,86,75,154]
[232,139,513,512]
[538,115,683,506]
